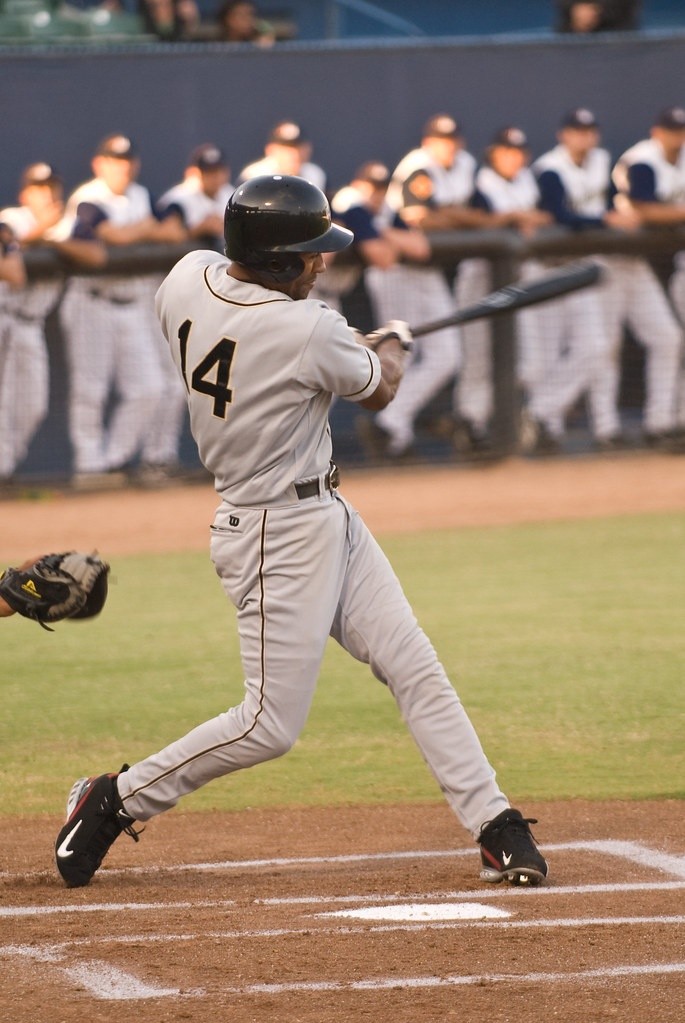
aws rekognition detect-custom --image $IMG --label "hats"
[652,104,685,128]
[92,132,142,160]
[421,114,462,138]
[185,142,231,174]
[18,163,65,194]
[490,126,531,152]
[560,108,602,130]
[355,160,391,188]
[269,119,312,148]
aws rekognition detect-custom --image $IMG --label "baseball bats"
[412,264,599,337]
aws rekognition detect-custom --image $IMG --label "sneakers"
[55,764,146,888]
[476,808,549,887]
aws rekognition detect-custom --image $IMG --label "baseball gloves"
[1,550,111,622]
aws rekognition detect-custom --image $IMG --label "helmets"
[225,175,354,284]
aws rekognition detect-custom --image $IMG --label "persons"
[0,550,111,631]
[309,164,431,318]
[0,164,107,483]
[609,110,685,446]
[54,174,549,885]
[60,137,183,483]
[236,124,330,199]
[555,0,643,36]
[50,0,289,48]
[538,112,641,445]
[457,126,556,459]
[138,146,239,479]
[366,116,487,453]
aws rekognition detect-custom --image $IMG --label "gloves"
[365,320,413,351]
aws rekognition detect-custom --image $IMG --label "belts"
[294,459,341,499]
